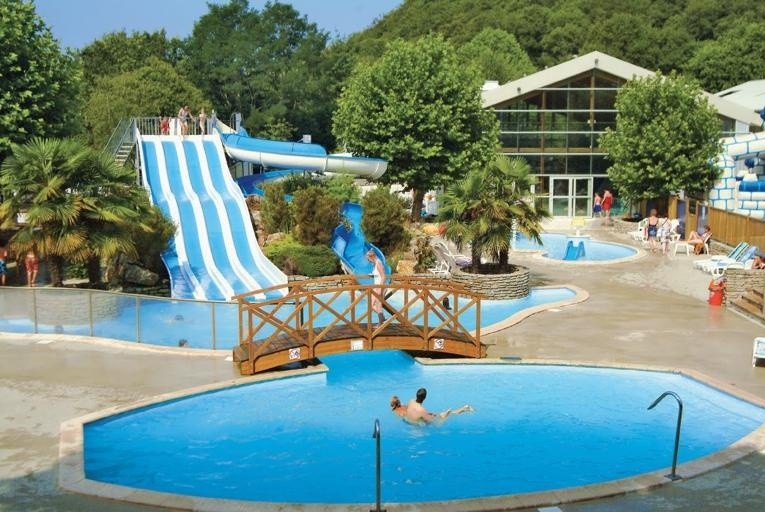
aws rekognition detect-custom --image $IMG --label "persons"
[0,238,10,286]
[402,387,437,427]
[645,209,662,253]
[675,224,712,247]
[159,115,169,136]
[439,297,458,311]
[600,187,614,225]
[365,250,387,328]
[439,221,446,240]
[178,339,189,347]
[198,107,206,135]
[170,315,184,324]
[177,105,195,135]
[660,213,672,256]
[456,233,464,254]
[391,396,475,420]
[420,194,432,217]
[751,254,765,270]
[592,192,603,218]
[24,249,39,287]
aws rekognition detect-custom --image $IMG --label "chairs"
[711,252,765,276]
[628,218,712,257]
[694,241,749,270]
[702,246,759,273]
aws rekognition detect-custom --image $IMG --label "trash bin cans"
[708,279,725,305]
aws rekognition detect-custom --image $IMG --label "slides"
[135,128,289,301]
[221,127,391,288]
[562,240,585,260]
